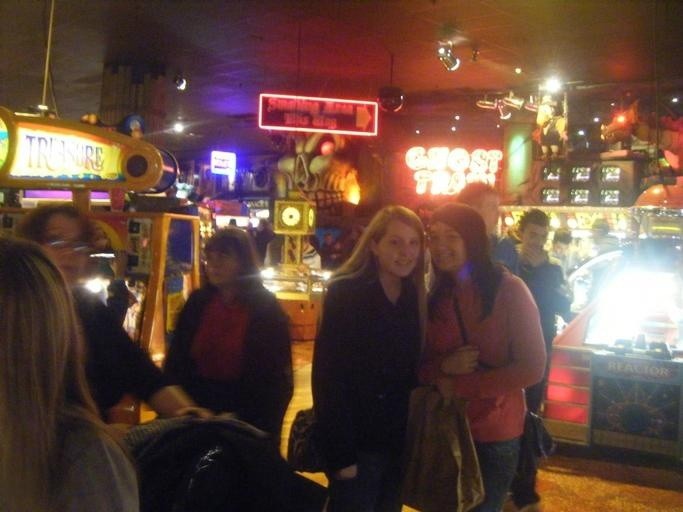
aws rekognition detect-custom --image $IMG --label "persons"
[229,220,361,271]
[546,217,631,275]
[286,203,432,510]
[1,238,140,512]
[158,226,293,446]
[511,209,576,512]
[458,181,541,512]
[21,204,215,428]
[409,203,549,511]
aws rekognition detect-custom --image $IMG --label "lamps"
[376,54,405,112]
[475,87,559,122]
[172,69,188,92]
[434,30,465,73]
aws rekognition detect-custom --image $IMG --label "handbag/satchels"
[287,407,325,472]
[524,410,556,458]
[401,387,485,512]
